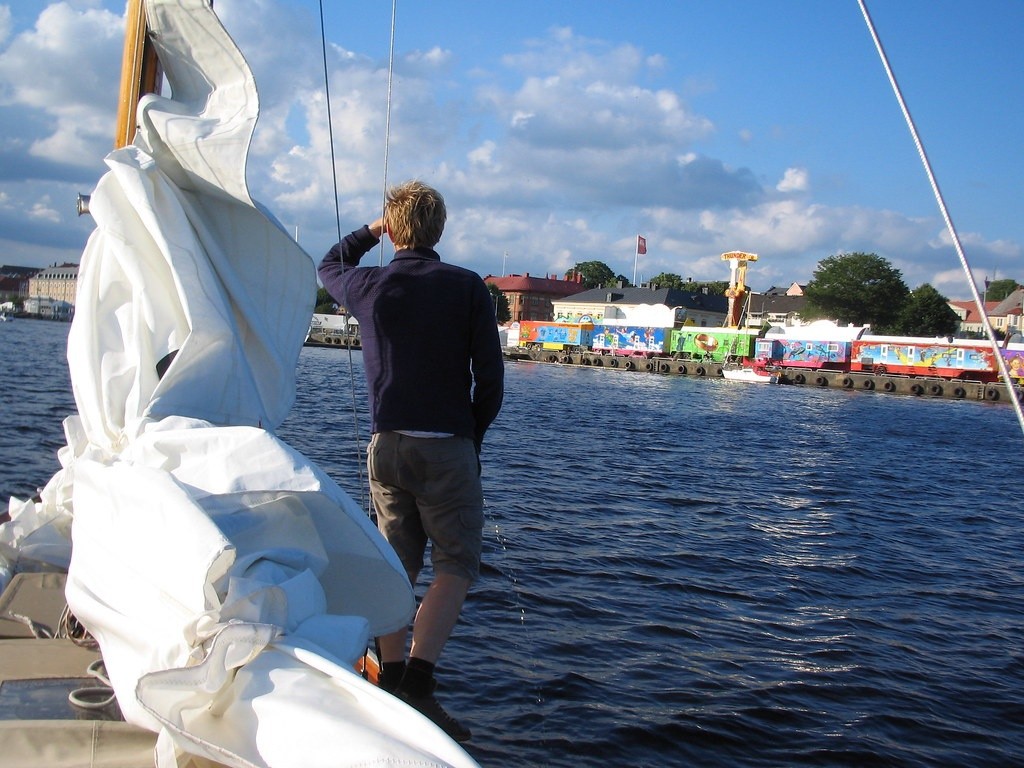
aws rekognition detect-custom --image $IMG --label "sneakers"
[376,669,404,693]
[392,666,472,741]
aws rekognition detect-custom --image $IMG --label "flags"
[638,235,646,254]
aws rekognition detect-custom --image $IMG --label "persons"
[319,177,503,743]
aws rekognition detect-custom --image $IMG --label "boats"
[0,311,14,322]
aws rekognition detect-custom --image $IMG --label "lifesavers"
[883,381,895,392]
[325,336,359,345]
[1011,390,1024,405]
[841,377,854,388]
[547,354,572,364]
[645,362,653,371]
[815,376,825,386]
[715,366,724,377]
[953,387,966,399]
[985,388,1000,401]
[581,357,633,370]
[794,374,804,384]
[659,362,706,376]
[909,384,944,397]
[862,379,874,390]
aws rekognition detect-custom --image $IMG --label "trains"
[517,321,1024,387]
